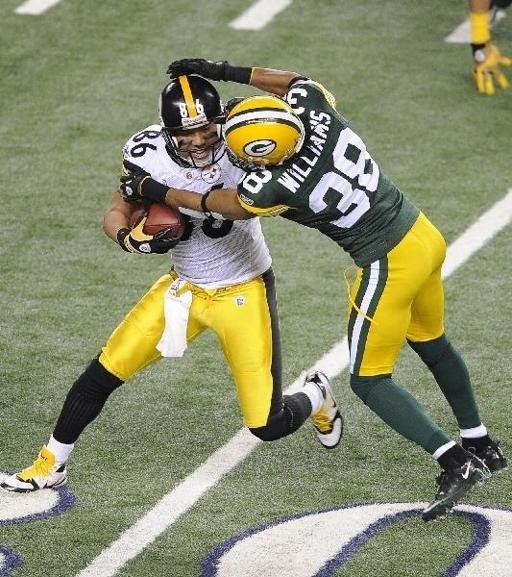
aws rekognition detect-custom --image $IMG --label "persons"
[0,75,344,493]
[463,0,512,95]
[118,57,510,523]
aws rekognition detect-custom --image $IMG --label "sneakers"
[422,456,489,521]
[304,370,343,450]
[0,445,73,495]
[461,438,508,472]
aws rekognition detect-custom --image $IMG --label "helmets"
[160,75,221,160]
[221,95,305,171]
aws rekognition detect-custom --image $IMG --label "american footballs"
[127,203,183,239]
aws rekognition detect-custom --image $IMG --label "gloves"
[166,57,231,82]
[470,44,512,97]
[123,215,180,254]
[119,160,152,201]
